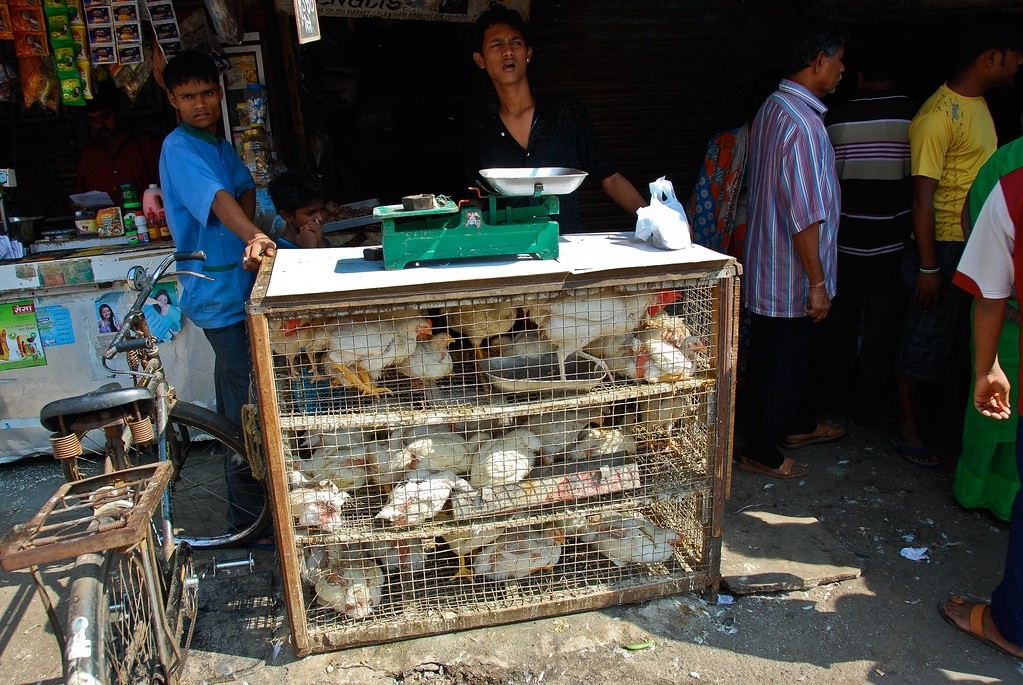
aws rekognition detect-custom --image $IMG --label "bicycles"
[0,249,269,685]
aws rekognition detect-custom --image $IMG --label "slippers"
[888,436,940,466]
[785,424,843,450]
[737,455,807,479]
[938,597,1023,661]
[240,535,278,551]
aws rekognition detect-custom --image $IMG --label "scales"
[0,168,38,247]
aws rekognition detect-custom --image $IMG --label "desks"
[0,238,218,465]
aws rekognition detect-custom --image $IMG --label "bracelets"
[242,256,250,263]
[247,236,270,246]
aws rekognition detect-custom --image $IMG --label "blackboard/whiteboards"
[294,0,321,44]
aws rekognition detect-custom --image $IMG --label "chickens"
[268,291,710,401]
[286,394,696,620]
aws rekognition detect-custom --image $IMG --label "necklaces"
[530,105,541,134]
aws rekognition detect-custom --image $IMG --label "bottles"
[147,212,161,242]
[134,211,150,243]
[142,184,165,221]
[159,211,173,241]
[120,183,143,244]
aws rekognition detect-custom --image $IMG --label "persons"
[952,136,1023,521]
[73,84,160,204]
[97,304,123,333]
[819,28,917,425]
[158,49,277,549]
[272,168,332,249]
[684,89,771,458]
[887,12,1023,465]
[939,165,1023,662]
[456,4,650,234]
[153,289,180,334]
[736,16,841,476]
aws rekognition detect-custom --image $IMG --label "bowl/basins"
[0,216,48,246]
[478,352,607,394]
